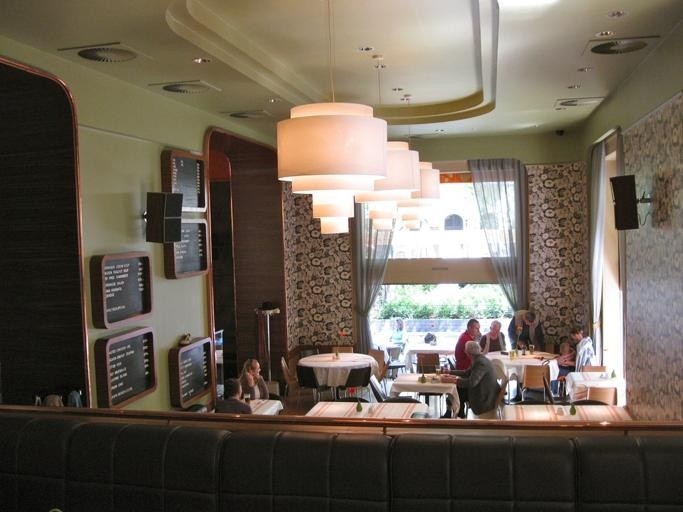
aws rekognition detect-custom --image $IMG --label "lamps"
[276,0,444,235]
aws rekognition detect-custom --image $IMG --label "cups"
[430,365,442,384]
[556,408,563,415]
[243,393,250,405]
[506,343,535,359]
[600,372,611,381]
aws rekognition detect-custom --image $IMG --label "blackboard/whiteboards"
[161,150,208,212]
[95,327,158,409]
[90,251,154,329]
[168,336,214,408]
[164,219,208,279]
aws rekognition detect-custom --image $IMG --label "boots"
[457,409,465,417]
[441,412,451,418]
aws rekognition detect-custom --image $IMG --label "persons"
[420,332,439,351]
[385,316,406,353]
[453,319,490,370]
[213,377,251,416]
[508,309,544,352]
[478,320,506,354]
[237,357,270,401]
[551,325,596,400]
[438,340,500,419]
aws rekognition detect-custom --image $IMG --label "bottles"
[421,374,425,383]
[356,400,361,411]
[569,404,576,415]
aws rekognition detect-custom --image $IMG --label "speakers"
[610,175,639,231]
[146,192,183,245]
